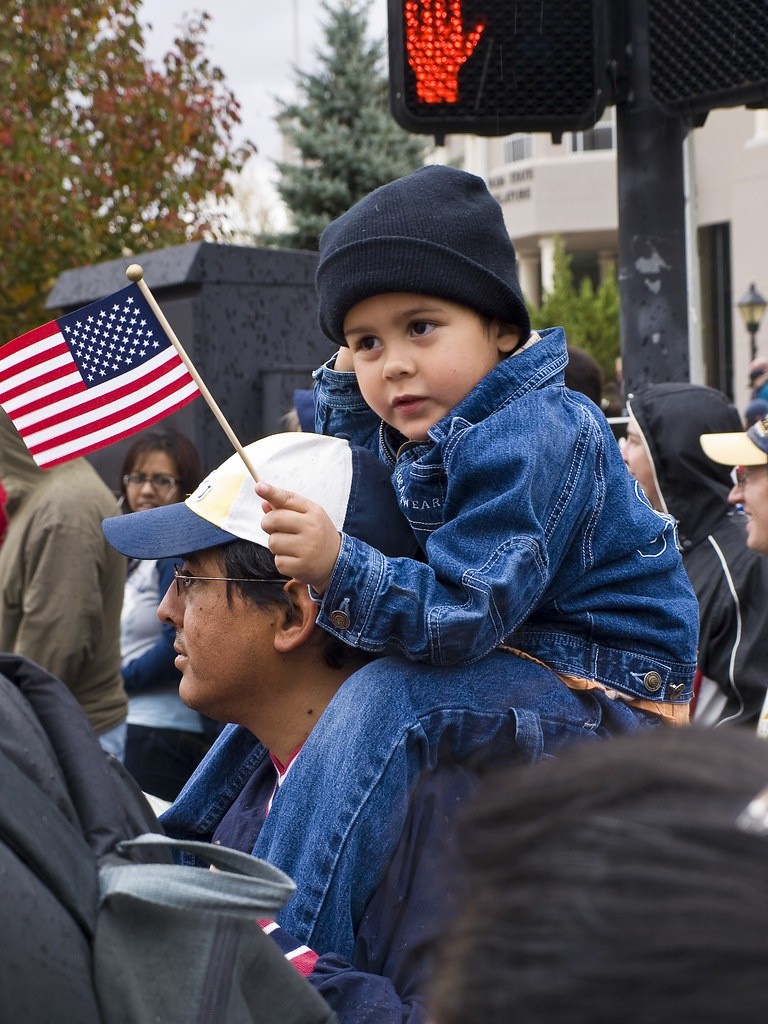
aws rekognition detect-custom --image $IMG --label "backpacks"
[0,755,339,1024]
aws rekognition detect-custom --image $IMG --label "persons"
[119,431,212,803]
[0,396,128,772]
[249,161,700,966]
[418,349,768,1024]
[101,431,490,1023]
[282,381,316,434]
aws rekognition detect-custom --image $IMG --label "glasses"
[123,472,180,492]
[172,561,289,597]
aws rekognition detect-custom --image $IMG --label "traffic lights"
[632,0,768,109]
[386,1,611,140]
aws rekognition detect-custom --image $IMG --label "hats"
[700,409,768,466]
[314,165,533,356]
[99,432,428,564]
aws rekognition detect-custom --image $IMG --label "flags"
[0,281,203,471]
